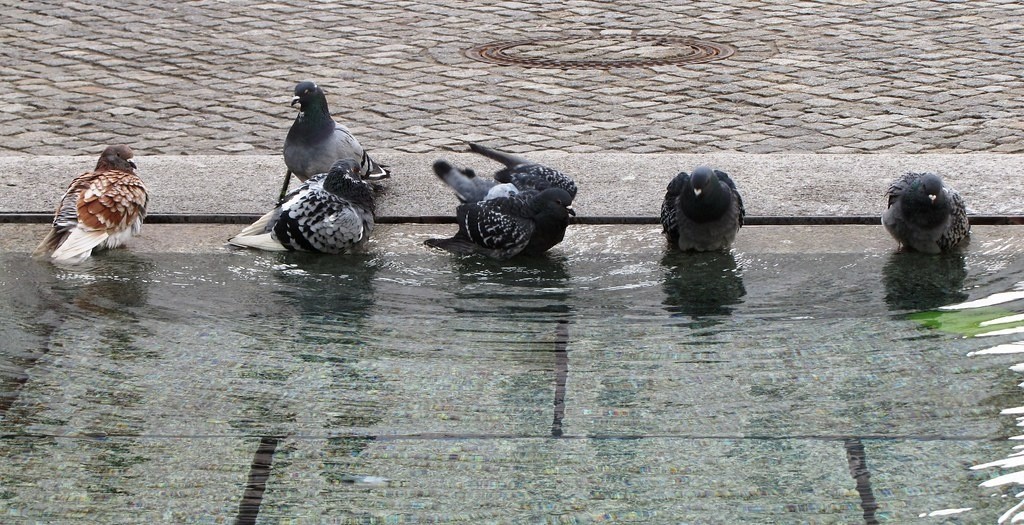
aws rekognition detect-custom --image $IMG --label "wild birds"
[32,144,151,267]
[661,166,746,254]
[283,81,391,182]
[227,157,390,257]
[880,172,971,255]
[423,140,577,267]
